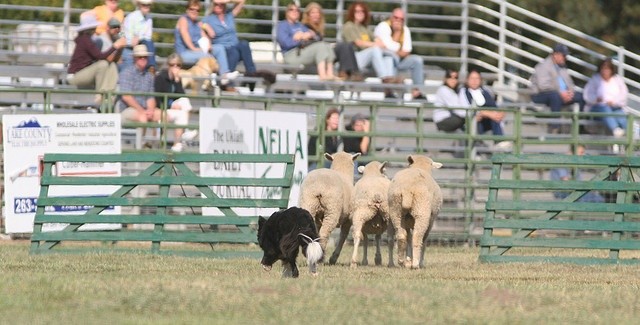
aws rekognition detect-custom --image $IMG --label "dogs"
[257,206,324,278]
[180,56,220,91]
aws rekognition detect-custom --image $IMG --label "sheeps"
[388,154,443,270]
[348,161,395,270]
[299,151,361,266]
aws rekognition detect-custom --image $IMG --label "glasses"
[139,3,152,7]
[110,25,121,30]
[135,56,148,59]
[168,63,182,67]
[188,7,199,13]
[288,8,300,12]
[393,15,404,22]
[446,74,458,80]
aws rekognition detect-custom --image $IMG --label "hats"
[554,44,572,55]
[345,114,366,130]
[76,12,103,32]
[132,44,153,57]
[107,17,120,28]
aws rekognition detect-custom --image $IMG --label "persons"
[82,0,125,35]
[342,112,372,179]
[93,18,139,71]
[459,69,505,147]
[119,0,155,73]
[581,58,629,151]
[308,108,343,172]
[341,3,402,99]
[302,2,324,43]
[528,44,585,134]
[66,16,118,103]
[276,0,342,82]
[154,53,198,153]
[550,144,605,204]
[199,1,258,92]
[432,67,473,133]
[173,1,239,86]
[374,7,427,100]
[119,44,169,150]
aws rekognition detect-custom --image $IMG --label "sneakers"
[181,130,199,141]
[413,93,428,103]
[171,142,183,152]
[383,92,402,103]
[221,71,240,86]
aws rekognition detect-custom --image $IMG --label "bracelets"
[202,22,207,30]
